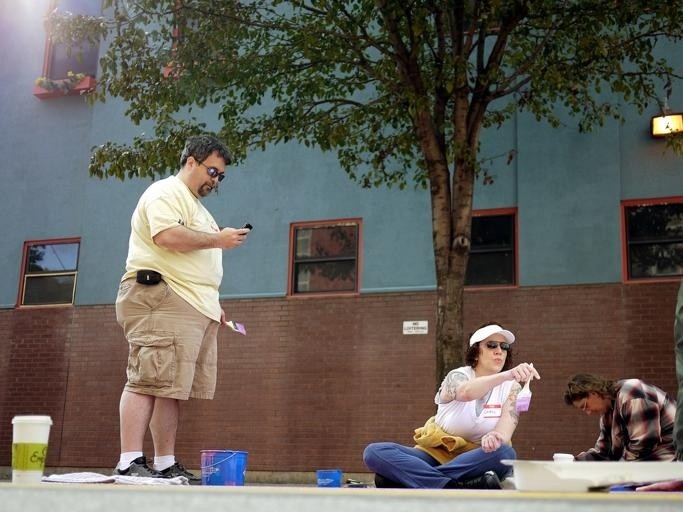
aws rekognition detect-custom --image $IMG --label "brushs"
[226,321,246,336]
[516,363,533,411]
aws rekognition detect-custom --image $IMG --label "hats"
[469,324,515,347]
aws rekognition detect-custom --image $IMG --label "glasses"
[486,340,510,351]
[198,159,225,182]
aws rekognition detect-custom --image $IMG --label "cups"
[552,452,574,464]
[11,414,52,481]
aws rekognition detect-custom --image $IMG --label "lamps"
[649,112,682,141]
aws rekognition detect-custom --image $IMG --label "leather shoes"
[464,469,503,489]
[374,472,392,488]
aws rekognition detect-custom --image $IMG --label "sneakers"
[155,460,202,486]
[112,455,165,481]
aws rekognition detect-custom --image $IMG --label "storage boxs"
[498,459,683,494]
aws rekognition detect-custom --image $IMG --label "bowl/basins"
[499,458,590,492]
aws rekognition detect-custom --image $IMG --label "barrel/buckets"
[199,449,248,487]
[316,469,344,488]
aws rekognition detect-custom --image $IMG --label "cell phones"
[238,222,252,231]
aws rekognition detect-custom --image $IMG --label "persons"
[563,372,677,461]
[362,320,532,489]
[112,134,251,484]
